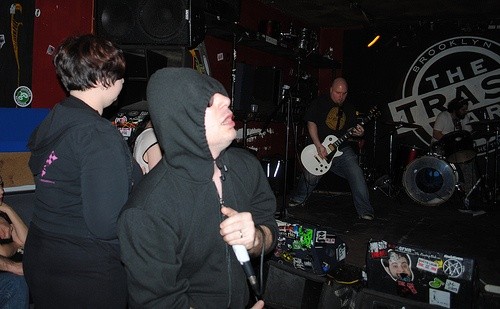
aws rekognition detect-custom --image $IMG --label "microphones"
[233,245,260,295]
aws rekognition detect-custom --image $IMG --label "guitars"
[301,105,381,176]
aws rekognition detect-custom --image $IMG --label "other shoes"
[284,202,299,218]
[360,215,373,224]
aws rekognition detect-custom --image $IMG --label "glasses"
[207,96,214,107]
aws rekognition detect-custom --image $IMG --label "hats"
[447,96,472,113]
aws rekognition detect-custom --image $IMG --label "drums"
[398,143,427,169]
[402,153,459,207]
[438,130,474,164]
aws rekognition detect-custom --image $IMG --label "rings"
[240,230,243,239]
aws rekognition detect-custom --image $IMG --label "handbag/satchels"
[333,238,365,274]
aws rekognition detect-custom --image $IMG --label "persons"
[0,176,30,309]
[383,251,414,282]
[116,68,279,309]
[127,114,162,175]
[22,32,144,309]
[433,97,477,193]
[286,77,375,220]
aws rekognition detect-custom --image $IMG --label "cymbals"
[383,122,422,129]
[466,121,496,125]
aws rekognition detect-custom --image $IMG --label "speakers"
[364,238,481,309]
[274,220,349,278]
[94,0,207,47]
